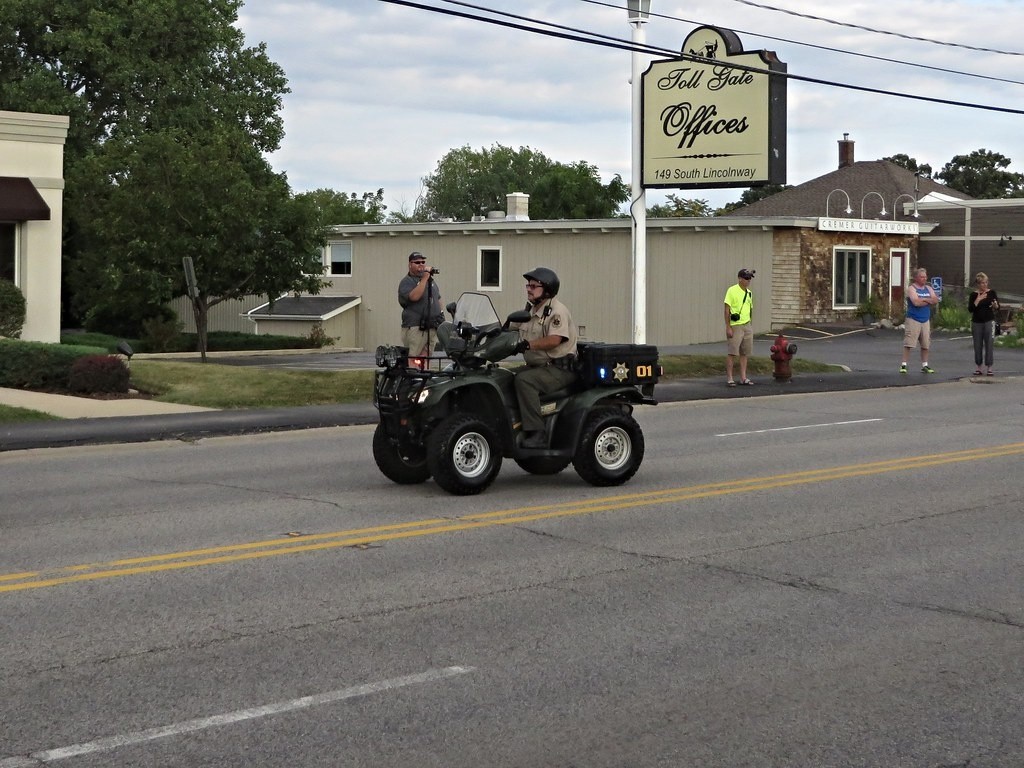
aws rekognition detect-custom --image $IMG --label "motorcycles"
[372,291,663,497]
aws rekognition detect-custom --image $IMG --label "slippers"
[726,380,736,387]
[739,378,754,385]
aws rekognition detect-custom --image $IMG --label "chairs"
[539,342,591,403]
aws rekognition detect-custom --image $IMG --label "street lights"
[628,0,651,346]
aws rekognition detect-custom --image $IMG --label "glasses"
[526,284,543,290]
[411,261,425,264]
[744,276,751,280]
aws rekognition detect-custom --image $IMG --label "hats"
[738,269,756,277]
[409,252,427,262]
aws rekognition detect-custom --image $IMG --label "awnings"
[0,176,50,221]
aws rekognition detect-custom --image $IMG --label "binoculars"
[430,315,445,331]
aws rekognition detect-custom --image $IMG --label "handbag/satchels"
[430,312,445,330]
[995,323,1000,336]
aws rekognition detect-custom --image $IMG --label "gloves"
[510,339,530,356]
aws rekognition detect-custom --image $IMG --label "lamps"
[861,192,887,219]
[999,233,1012,246]
[826,189,853,217]
[894,194,919,220]
[117,340,133,361]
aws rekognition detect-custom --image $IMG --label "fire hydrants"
[768,333,798,383]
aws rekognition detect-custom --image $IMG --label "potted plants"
[853,295,879,326]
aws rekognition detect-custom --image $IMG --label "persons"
[898,268,939,374]
[503,267,580,449]
[724,269,756,387]
[398,251,444,369]
[968,272,1000,376]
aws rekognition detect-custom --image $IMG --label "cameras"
[731,313,739,321]
[430,269,440,276]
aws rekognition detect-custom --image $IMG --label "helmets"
[523,267,560,298]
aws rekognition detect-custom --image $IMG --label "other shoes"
[973,371,982,375]
[521,431,549,449]
[987,371,994,375]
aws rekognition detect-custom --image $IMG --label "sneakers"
[920,366,934,373]
[899,365,907,373]
